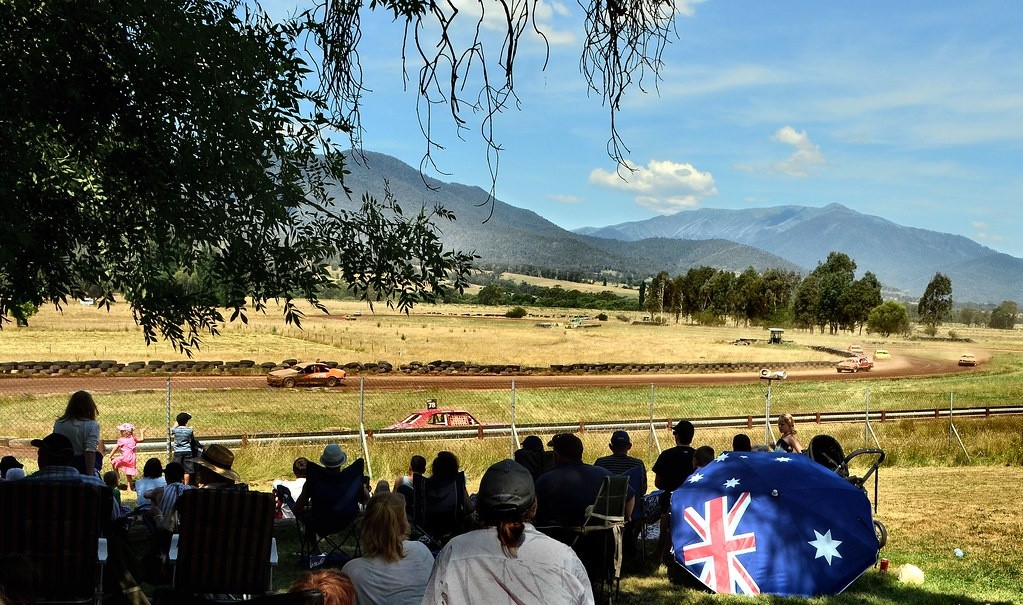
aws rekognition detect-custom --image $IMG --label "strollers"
[799,435,887,549]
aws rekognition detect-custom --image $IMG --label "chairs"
[537,475,630,605]
[179,489,275,605]
[410,472,465,559]
[0,480,113,605]
[285,458,367,568]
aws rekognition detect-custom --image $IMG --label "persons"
[0,389,802,604]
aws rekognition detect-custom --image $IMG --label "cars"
[266,362,347,388]
[342,311,362,320]
[848,345,864,355]
[958,353,978,368]
[378,408,509,434]
[873,348,893,361]
[835,356,873,373]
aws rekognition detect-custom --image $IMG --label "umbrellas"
[668,450,882,597]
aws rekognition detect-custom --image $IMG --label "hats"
[670,420,694,437]
[520,433,584,456]
[161,462,185,478]
[478,459,536,518]
[117,423,135,432]
[319,443,347,468]
[0,456,25,481]
[186,444,240,480]
[30,433,75,460]
[176,412,192,422]
[611,430,629,446]
